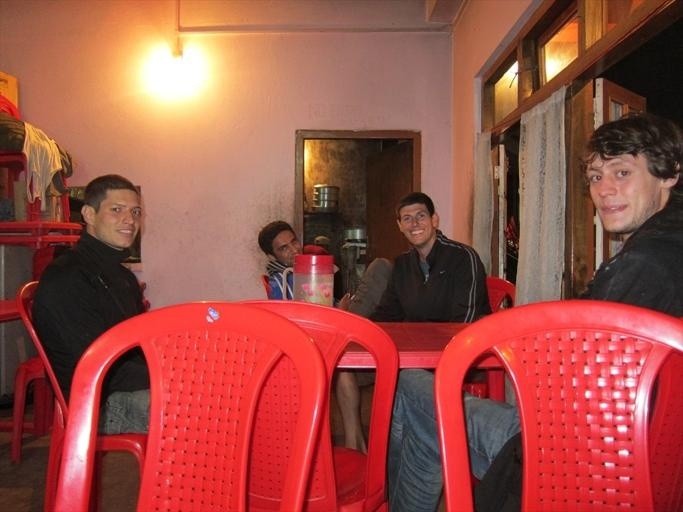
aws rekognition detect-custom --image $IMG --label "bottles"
[48,193,64,222]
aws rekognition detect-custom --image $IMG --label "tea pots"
[283,254,339,307]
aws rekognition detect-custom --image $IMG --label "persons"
[385,111,683,512]
[335,190,493,457]
[31,172,151,434]
[258,220,393,318]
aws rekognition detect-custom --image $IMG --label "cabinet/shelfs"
[0,97,80,462]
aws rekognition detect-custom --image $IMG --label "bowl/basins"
[345,225,367,241]
